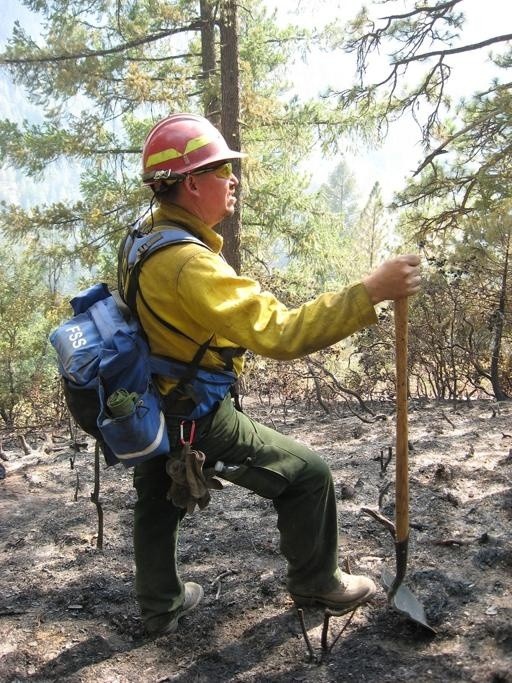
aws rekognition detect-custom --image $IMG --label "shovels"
[381,294,437,639]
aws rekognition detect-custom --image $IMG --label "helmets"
[139,113,247,186]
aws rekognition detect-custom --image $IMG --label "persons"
[119,111,422,639]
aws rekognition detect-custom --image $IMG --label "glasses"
[191,163,233,179]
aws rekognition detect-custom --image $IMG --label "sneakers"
[286,566,376,610]
[145,581,205,634]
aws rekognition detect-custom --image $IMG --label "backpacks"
[49,209,239,469]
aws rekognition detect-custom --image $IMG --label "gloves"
[166,448,224,515]
